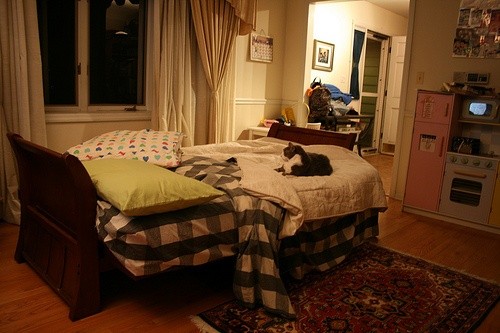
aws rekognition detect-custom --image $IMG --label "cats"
[274,142,333,178]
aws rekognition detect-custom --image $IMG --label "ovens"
[437,153,500,225]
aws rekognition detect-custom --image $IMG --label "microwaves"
[452,136,480,154]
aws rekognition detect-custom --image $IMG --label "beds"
[6,122,389,322]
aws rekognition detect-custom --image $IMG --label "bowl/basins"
[307,122,321,129]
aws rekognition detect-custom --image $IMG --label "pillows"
[81,158,227,218]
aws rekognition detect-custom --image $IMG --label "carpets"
[189,241,500,333]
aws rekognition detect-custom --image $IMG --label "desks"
[248,125,270,139]
[335,114,376,145]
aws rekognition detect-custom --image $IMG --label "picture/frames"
[311,39,335,72]
